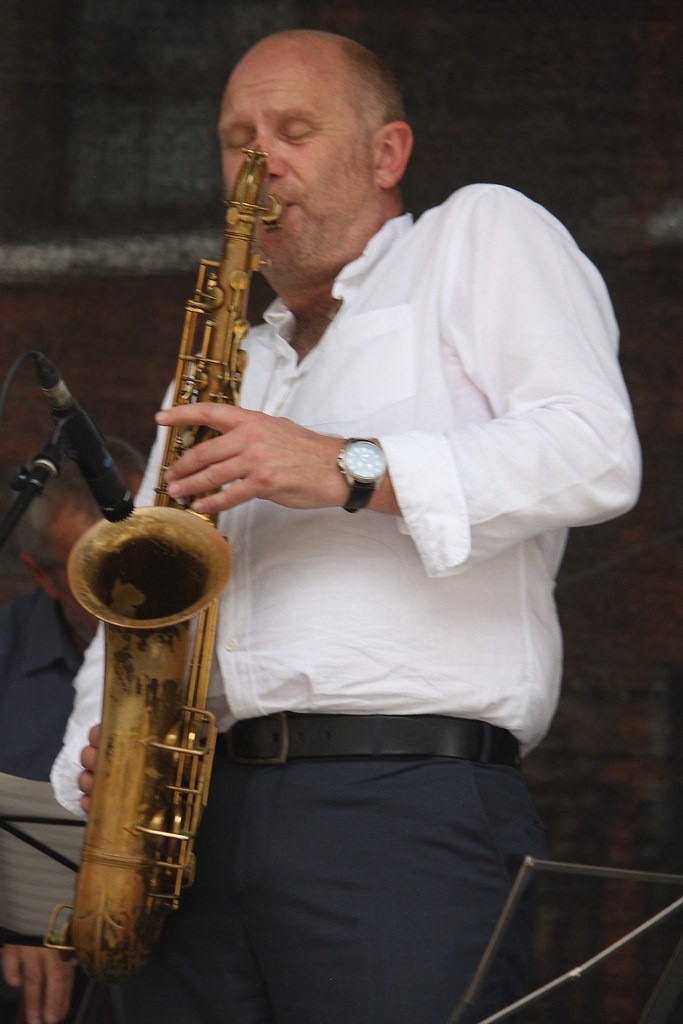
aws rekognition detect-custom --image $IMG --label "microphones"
[33,352,136,523]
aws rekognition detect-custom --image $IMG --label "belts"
[216,709,519,765]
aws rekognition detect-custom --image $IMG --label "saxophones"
[44,146,282,984]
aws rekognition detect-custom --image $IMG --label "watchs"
[336,437,386,516]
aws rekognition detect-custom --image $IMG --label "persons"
[49,28,643,1024]
[0,436,161,1024]
